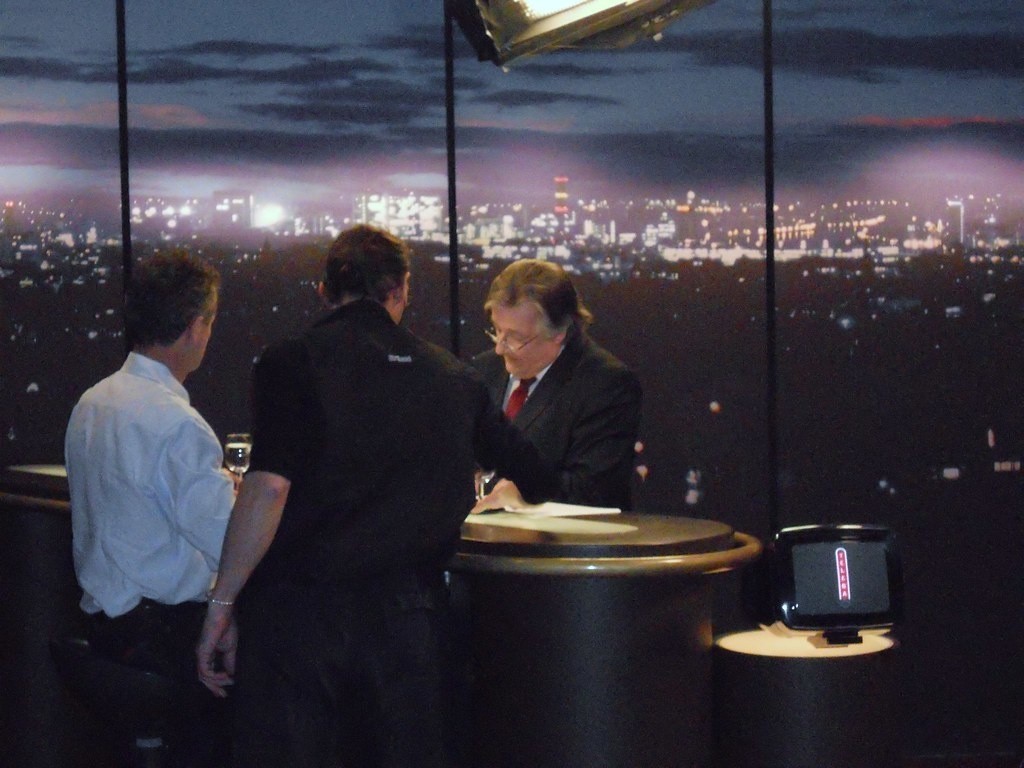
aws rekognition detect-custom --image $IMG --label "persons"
[465,259,641,513]
[64,253,240,768]
[195,227,540,768]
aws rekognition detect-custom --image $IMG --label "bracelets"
[206,589,234,605]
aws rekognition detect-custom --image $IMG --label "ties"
[504,377,537,423]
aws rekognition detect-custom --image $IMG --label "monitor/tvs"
[773,525,904,629]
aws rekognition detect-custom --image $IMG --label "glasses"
[484,324,549,353]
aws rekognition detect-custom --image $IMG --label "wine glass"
[224,432,253,490]
